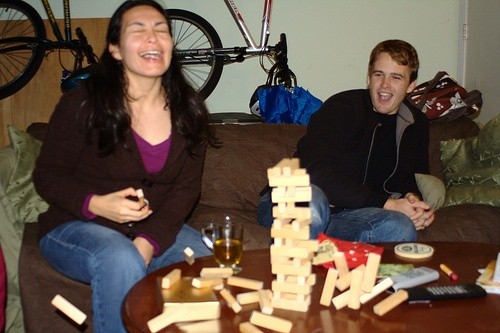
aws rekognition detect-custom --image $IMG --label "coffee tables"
[117,239,500,333]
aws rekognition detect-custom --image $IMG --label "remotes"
[404,283,487,301]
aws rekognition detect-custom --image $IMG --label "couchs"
[13,124,500,333]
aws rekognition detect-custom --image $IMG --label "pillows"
[441,113,500,213]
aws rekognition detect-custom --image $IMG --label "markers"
[439,263,458,280]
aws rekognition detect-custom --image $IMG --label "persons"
[33,0,217,333]
[257,39,435,242]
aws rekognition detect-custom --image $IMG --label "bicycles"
[163,0,297,104]
[0,0,100,101]
